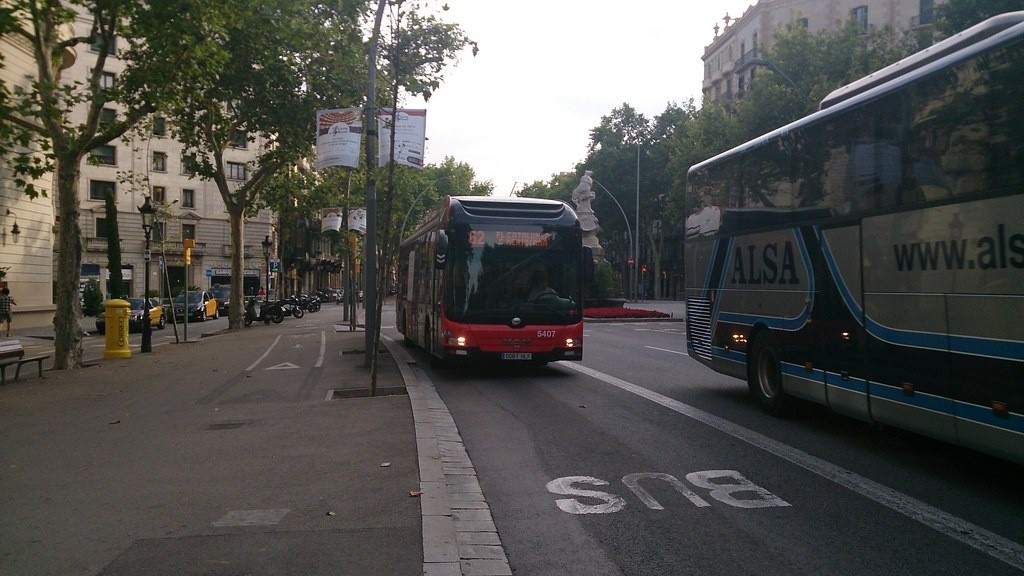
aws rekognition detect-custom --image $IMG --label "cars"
[168,289,220,322]
[208,284,245,316]
[94,297,167,335]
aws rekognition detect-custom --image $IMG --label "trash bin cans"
[102,300,131,359]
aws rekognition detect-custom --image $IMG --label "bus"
[684,9,1024,474]
[391,193,585,379]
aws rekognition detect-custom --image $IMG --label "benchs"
[0,339,51,386]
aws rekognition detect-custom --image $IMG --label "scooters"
[242,293,321,326]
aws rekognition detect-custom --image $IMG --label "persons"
[269,288,276,294]
[259,287,265,295]
[0,288,18,337]
[572,170,593,210]
[527,272,559,303]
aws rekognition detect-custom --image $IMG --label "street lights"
[136,196,157,354]
[261,235,273,302]
